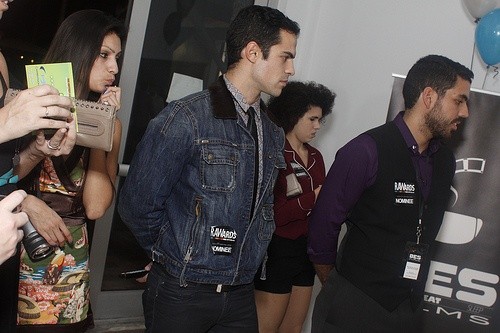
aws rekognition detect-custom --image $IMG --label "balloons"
[464,0,500,65]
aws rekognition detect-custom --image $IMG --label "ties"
[247,106,259,221]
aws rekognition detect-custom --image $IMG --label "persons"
[251,82,336,333]
[308,54,474,333]
[0,0,77,333]
[0,190,29,266]
[117,5,301,333]
[16,8,123,333]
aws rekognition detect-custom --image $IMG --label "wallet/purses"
[3,87,117,152]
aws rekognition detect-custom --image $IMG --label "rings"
[45,107,48,117]
[48,140,57,150]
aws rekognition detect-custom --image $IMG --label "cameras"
[13,204,55,260]
